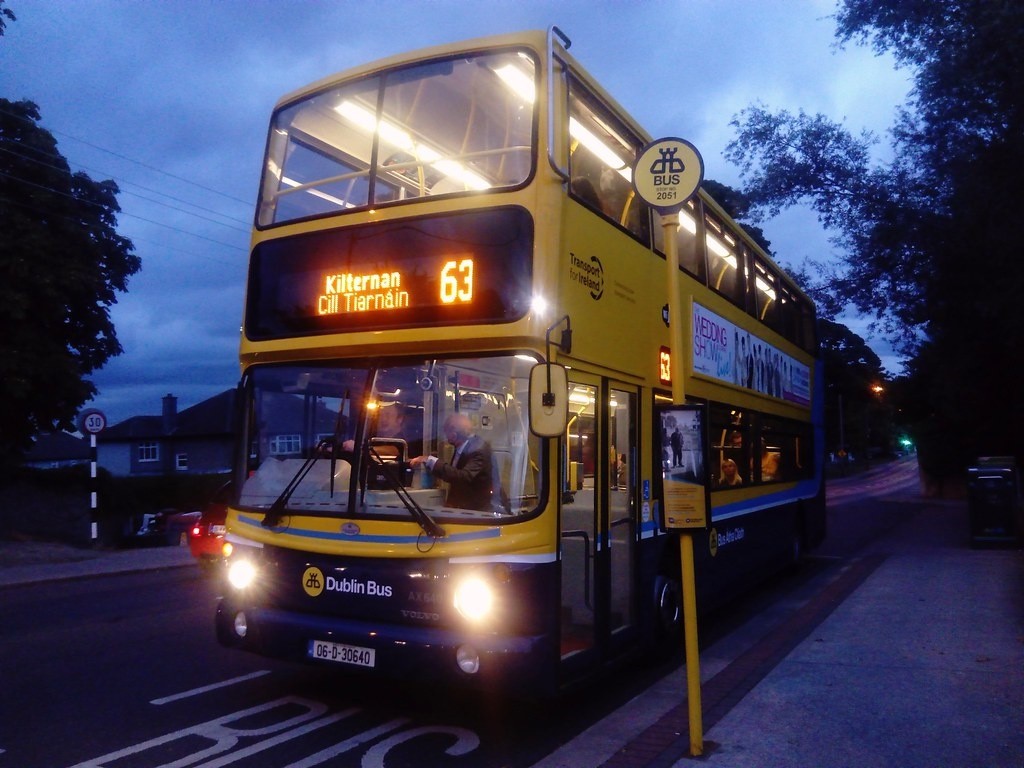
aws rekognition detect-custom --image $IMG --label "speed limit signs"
[84,413,105,434]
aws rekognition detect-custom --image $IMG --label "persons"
[732,331,789,400]
[663,427,684,473]
[313,398,414,490]
[717,431,783,484]
[407,414,502,512]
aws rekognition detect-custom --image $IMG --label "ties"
[450,449,457,467]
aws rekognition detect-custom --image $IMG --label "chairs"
[572,176,602,212]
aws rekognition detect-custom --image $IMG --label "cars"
[147,473,257,579]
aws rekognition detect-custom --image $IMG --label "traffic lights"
[899,436,912,450]
[869,381,885,395]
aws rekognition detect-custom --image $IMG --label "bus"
[215,23,828,709]
